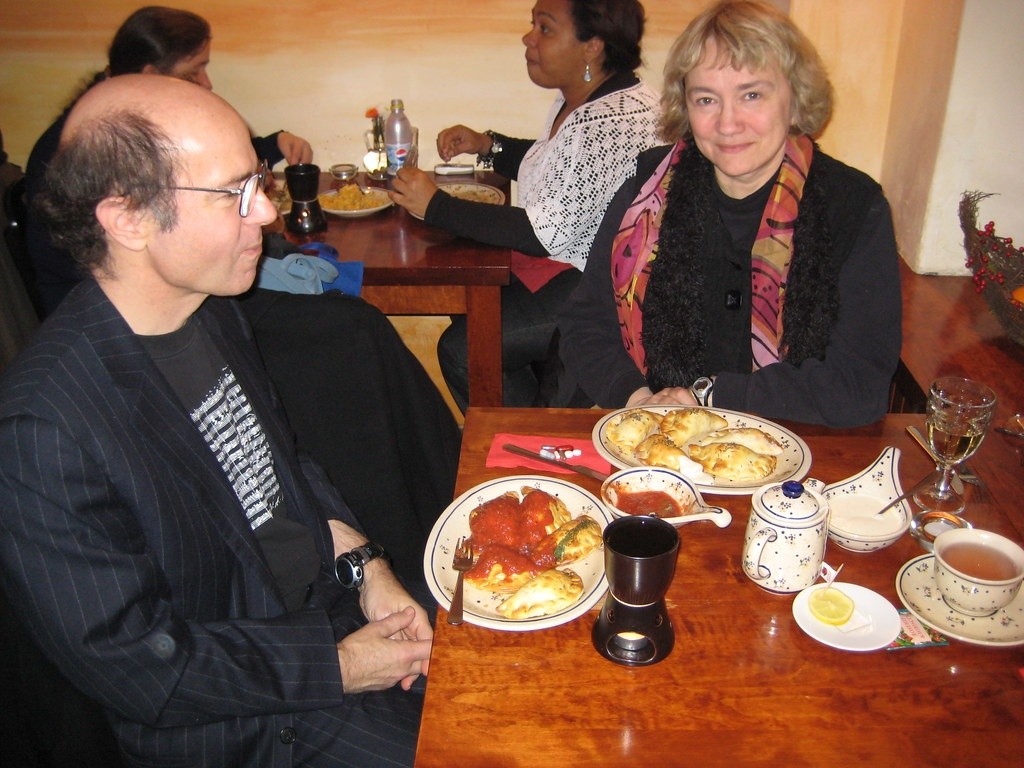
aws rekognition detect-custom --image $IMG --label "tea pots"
[741,478,830,595]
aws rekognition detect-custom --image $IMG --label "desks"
[254,167,515,405]
[413,403,1024,768]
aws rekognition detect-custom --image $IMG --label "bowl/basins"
[329,164,358,180]
[933,528,1024,616]
[601,466,733,528]
[821,446,912,553]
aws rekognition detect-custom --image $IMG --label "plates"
[267,180,292,215]
[792,582,902,652]
[423,475,615,631]
[592,404,812,496]
[317,186,394,218]
[896,553,1024,647]
[407,181,505,220]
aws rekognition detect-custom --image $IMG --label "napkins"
[484,433,612,479]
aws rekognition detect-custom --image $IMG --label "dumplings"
[464,486,602,620]
[607,407,784,482]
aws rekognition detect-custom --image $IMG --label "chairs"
[4,174,48,324]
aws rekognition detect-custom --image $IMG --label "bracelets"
[477,130,500,169]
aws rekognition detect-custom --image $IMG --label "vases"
[362,131,388,181]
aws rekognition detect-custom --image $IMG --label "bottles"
[387,99,412,189]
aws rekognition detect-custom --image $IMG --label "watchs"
[690,376,713,408]
[333,540,384,592]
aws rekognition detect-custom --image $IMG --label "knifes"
[906,425,965,495]
[504,444,609,482]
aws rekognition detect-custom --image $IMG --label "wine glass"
[913,376,997,514]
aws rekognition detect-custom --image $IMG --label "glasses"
[121,159,268,219]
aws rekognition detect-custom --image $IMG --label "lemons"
[810,588,855,625]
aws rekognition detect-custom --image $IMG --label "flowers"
[364,108,385,152]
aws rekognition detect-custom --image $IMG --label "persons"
[389,0,674,417]
[23,6,212,323]
[0,62,435,768]
[555,0,902,430]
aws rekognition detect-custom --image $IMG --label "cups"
[592,515,681,666]
[285,164,327,235]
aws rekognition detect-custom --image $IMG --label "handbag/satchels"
[302,243,363,298]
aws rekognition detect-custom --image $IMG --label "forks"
[959,462,983,486]
[447,538,473,625]
